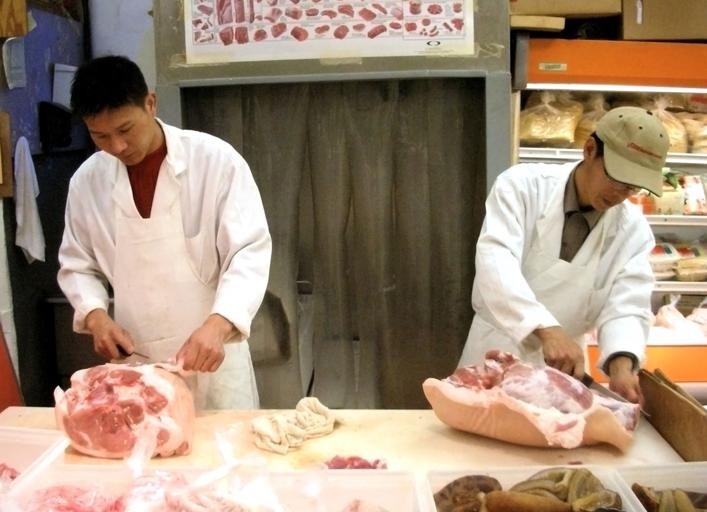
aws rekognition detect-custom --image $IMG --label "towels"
[15,137,46,266]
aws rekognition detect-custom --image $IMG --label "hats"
[594,106,670,198]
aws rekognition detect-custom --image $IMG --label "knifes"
[117,344,151,359]
[571,367,651,418]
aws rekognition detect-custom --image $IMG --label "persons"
[452,105,671,412]
[53,56,274,417]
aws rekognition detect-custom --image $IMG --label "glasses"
[598,149,641,195]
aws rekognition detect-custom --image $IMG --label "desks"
[0,406,686,467]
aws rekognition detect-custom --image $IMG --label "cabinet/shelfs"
[512,83,707,345]
[510,0,707,42]
